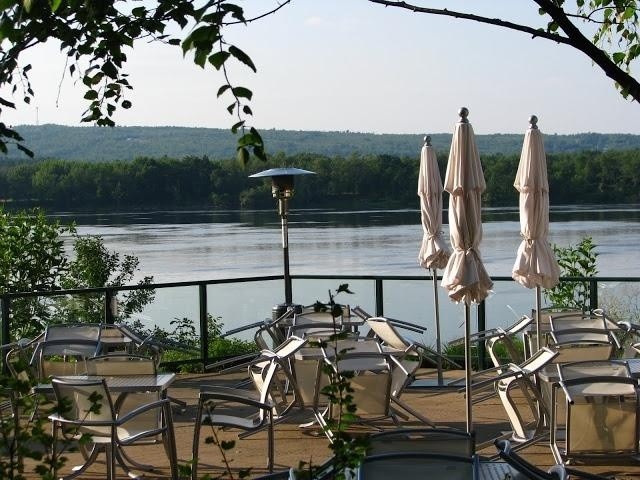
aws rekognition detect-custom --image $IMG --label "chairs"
[189,300,463,445]
[5,318,298,470]
[260,430,572,479]
[447,300,640,480]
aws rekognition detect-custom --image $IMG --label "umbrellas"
[417,107,494,433]
[512,115,560,389]
